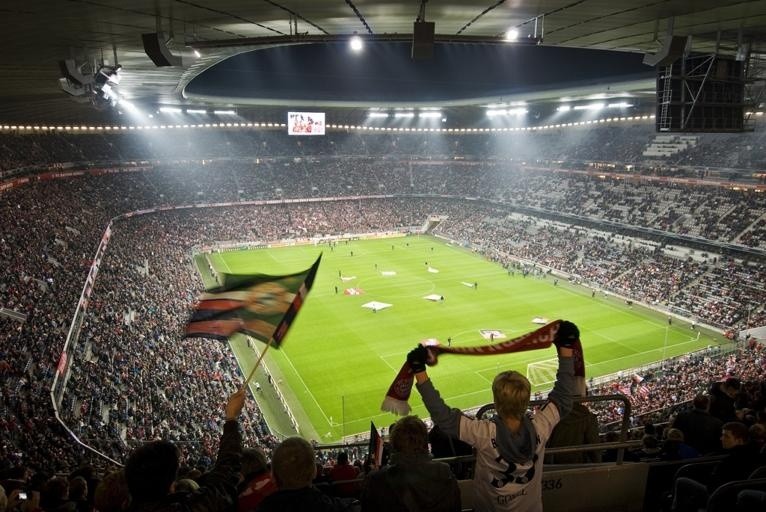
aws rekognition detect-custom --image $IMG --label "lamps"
[89,43,122,103]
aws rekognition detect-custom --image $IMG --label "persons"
[0,127,766,512]
[293,115,314,132]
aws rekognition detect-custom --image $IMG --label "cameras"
[14,492,33,501]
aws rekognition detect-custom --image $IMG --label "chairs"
[2,211,185,461]
[490,134,766,341]
